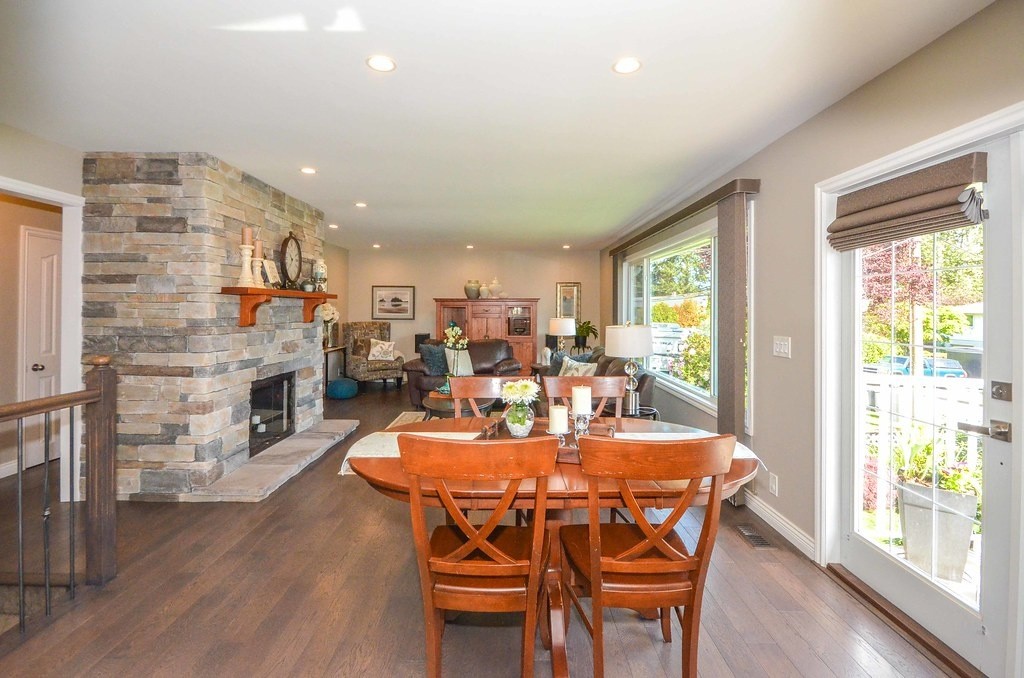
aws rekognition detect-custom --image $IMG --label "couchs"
[402,339,522,409]
[540,347,656,420]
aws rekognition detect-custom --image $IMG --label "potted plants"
[573,321,598,347]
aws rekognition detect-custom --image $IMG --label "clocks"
[280,231,302,289]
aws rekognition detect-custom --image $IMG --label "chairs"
[398,375,737,678]
[343,322,404,393]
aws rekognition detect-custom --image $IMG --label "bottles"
[464,280,482,299]
[300,279,316,292]
[489,279,503,298]
[479,284,489,298]
[312,259,328,293]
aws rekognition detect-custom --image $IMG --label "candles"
[572,383,592,414]
[252,415,266,433]
[241,228,263,258]
[548,402,568,433]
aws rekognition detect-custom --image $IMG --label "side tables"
[602,402,657,420]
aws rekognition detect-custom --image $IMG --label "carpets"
[385,411,503,429]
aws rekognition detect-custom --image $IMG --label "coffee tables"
[422,392,497,422]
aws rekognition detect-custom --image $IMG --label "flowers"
[890,422,981,498]
[443,324,469,350]
[320,303,340,326]
[500,378,543,424]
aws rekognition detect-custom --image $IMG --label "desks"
[338,416,768,527]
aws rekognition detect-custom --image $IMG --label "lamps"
[604,321,654,410]
[549,317,576,354]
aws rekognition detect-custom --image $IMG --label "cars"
[880,355,933,377]
[926,358,967,379]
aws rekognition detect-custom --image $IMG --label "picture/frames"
[263,260,281,285]
[372,285,415,320]
[556,281,580,323]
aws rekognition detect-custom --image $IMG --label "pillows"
[352,336,474,377]
[547,351,597,376]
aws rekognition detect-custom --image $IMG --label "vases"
[506,402,534,438]
[323,321,333,348]
[898,481,978,584]
[452,351,458,376]
[312,259,328,292]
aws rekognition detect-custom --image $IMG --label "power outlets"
[338,369,341,376]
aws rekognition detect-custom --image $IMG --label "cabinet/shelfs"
[434,298,538,376]
